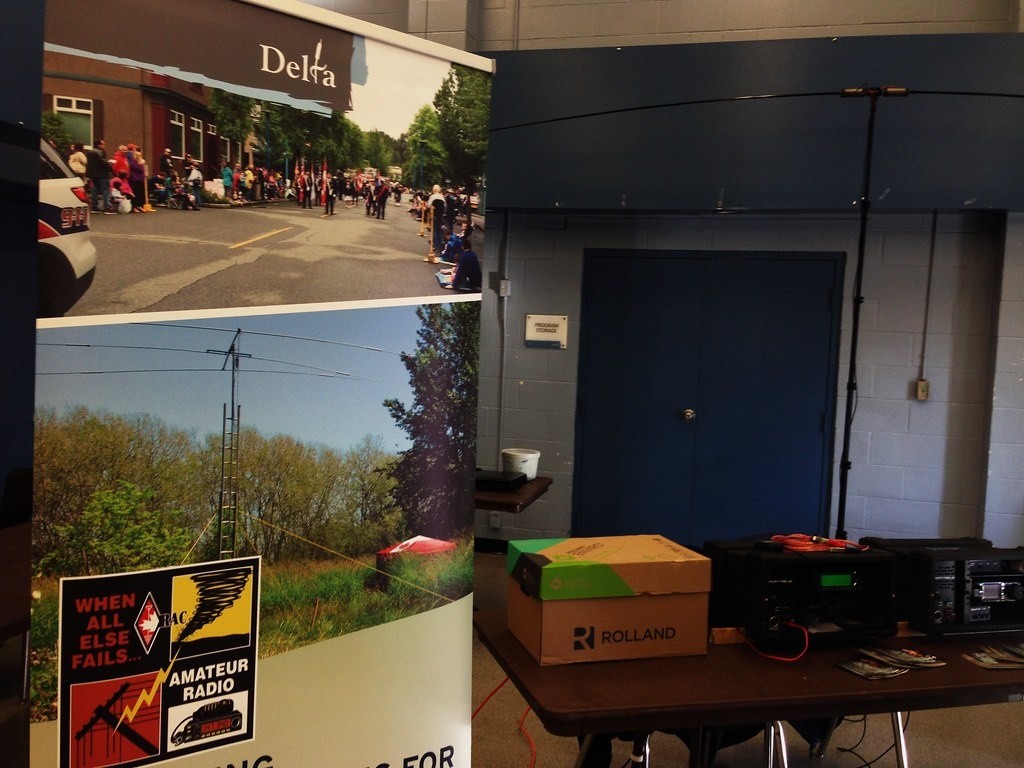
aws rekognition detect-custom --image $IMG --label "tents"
[375,533,456,593]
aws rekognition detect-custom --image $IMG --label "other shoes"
[132,207,147,213]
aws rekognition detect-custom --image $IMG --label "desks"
[473,605,1024,768]
[474,470,554,513]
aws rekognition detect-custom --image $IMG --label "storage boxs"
[505,531,712,667]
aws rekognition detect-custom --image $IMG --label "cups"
[502,448,540,481]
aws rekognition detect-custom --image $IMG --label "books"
[838,647,947,679]
[960,643,1024,668]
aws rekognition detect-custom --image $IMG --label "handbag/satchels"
[193,180,200,186]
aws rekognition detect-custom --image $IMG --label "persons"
[62,139,389,220]
[386,178,482,291]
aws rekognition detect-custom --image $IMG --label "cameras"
[744,601,808,647]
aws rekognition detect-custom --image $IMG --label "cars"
[39,138,97,318]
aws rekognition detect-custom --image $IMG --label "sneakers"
[91,208,102,213]
[104,208,118,214]
